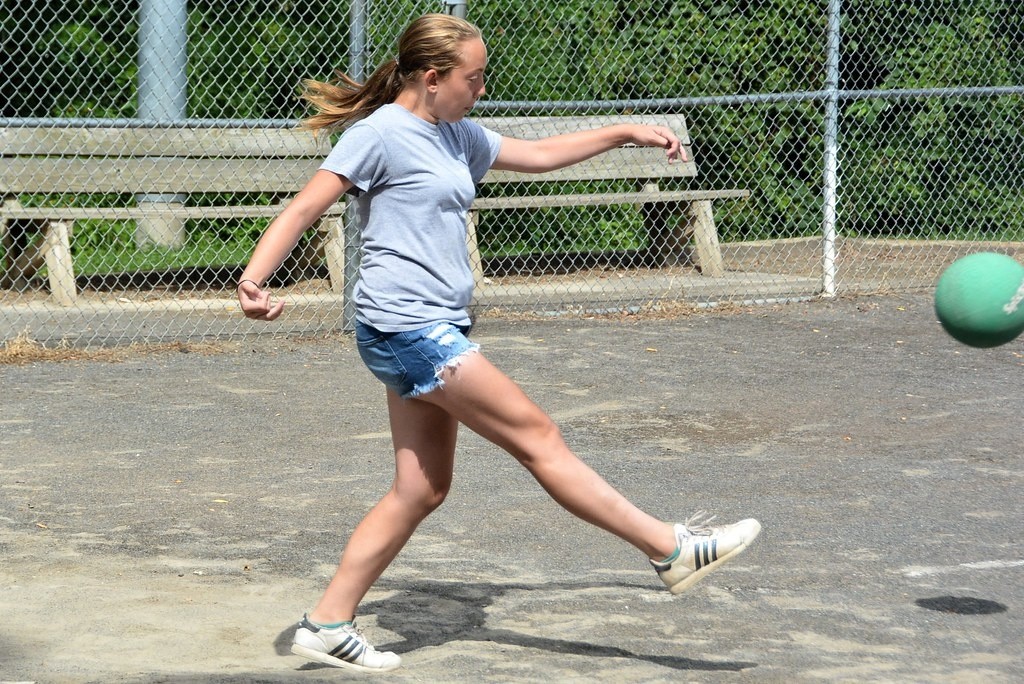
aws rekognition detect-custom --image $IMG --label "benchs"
[0,128,348,307]
[467,115,749,288]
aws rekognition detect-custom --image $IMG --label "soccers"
[934,253,1024,348]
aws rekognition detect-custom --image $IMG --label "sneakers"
[291,614,402,673]
[650,510,761,594]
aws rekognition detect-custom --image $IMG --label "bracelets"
[236,279,260,292]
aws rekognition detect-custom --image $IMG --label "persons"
[236,14,761,673]
